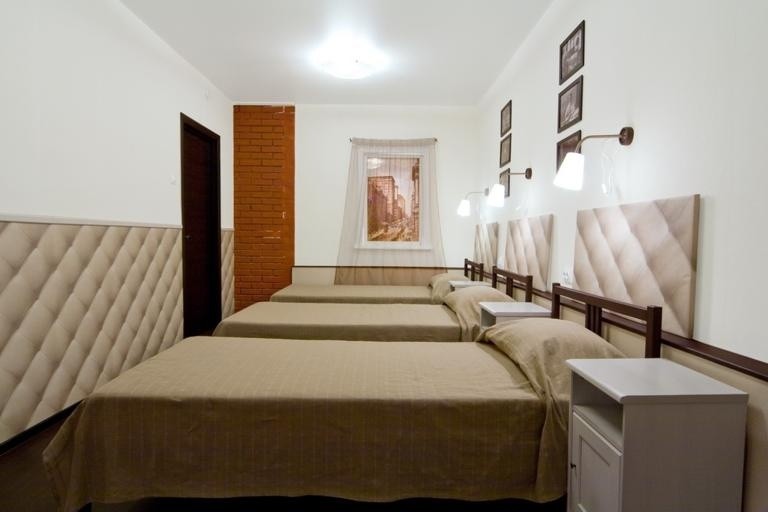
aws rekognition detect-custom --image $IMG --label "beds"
[269,258,483,303]
[43,283,662,512]
[212,266,533,341]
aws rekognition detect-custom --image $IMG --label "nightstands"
[449,280,493,291]
[478,302,552,333]
[565,358,750,511]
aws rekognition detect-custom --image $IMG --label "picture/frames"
[500,168,510,198]
[559,20,585,85]
[558,75,583,133]
[501,100,512,137]
[360,149,428,250]
[557,130,581,174]
[499,133,511,167]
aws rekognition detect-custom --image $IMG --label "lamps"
[553,127,633,191]
[486,169,531,207]
[457,188,489,217]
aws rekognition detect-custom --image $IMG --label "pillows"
[428,273,472,297]
[487,316,626,394]
[442,285,516,323]
